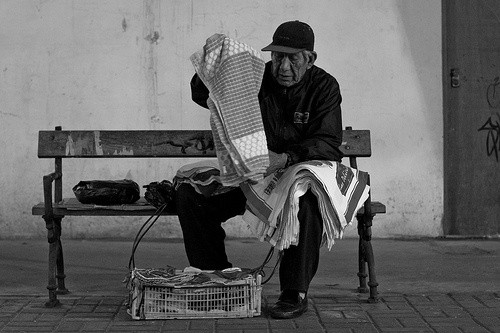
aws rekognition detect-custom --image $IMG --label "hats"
[261,20,314,53]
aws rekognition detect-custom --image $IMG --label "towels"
[172,149,292,197]
[190,32,270,187]
[239,160,371,253]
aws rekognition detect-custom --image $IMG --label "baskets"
[130,268,261,318]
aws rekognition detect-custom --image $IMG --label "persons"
[176,20,343,320]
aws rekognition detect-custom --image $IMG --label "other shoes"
[270,291,307,319]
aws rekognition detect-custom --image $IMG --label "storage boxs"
[132,267,263,319]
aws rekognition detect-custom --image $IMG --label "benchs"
[32,125,386,307]
